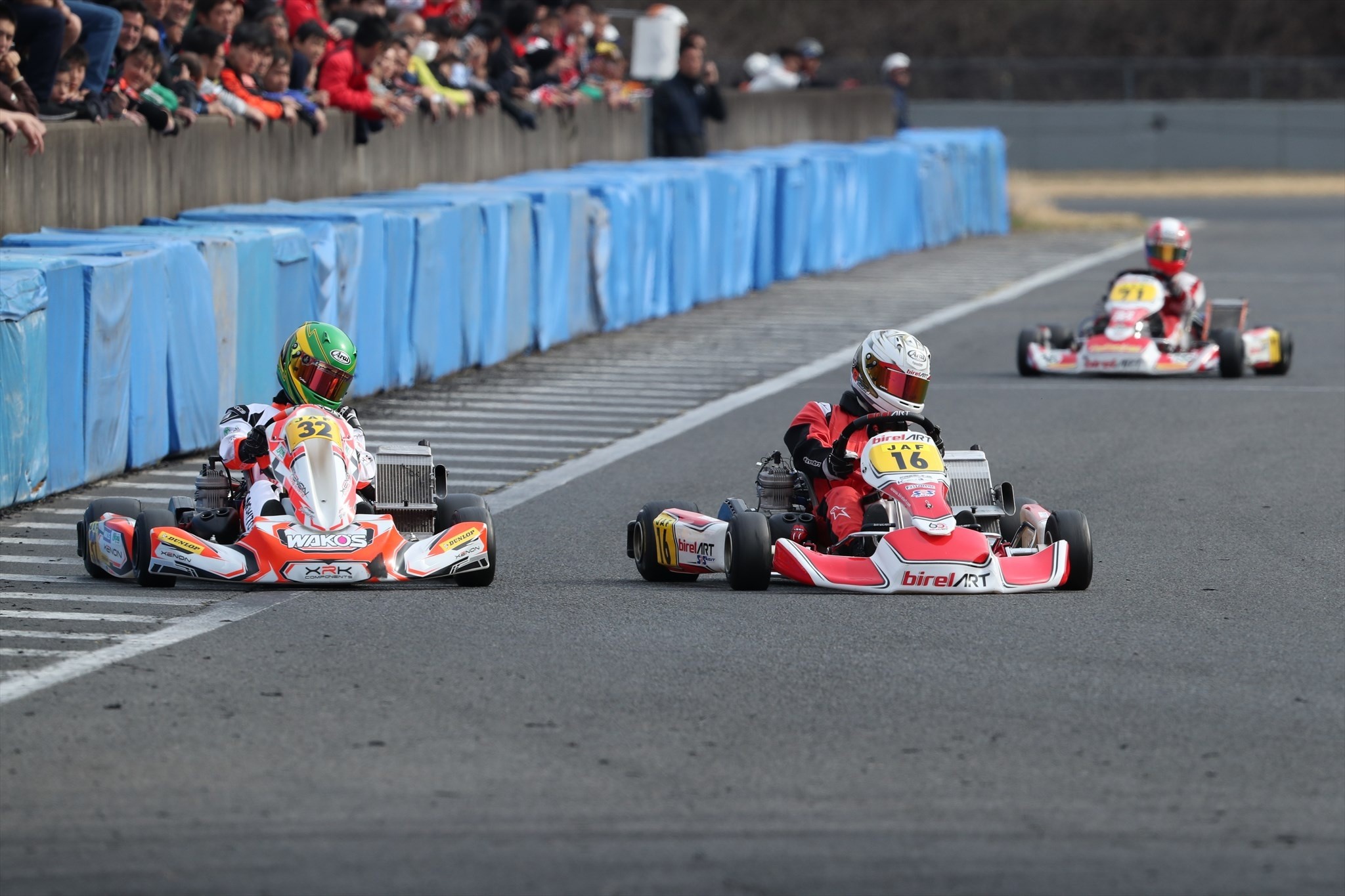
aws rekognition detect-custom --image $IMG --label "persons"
[783,330,978,557]
[881,53,912,128]
[1094,217,1206,353]
[0,0,859,156]
[219,321,376,532]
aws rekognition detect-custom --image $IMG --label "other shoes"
[355,501,375,515]
[955,510,976,530]
[861,503,891,556]
[1149,313,1169,351]
[32,99,77,120]
[261,499,286,516]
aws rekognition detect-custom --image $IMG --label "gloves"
[824,440,857,480]
[239,425,269,463]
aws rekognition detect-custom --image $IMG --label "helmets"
[791,35,825,59]
[1143,216,1192,279]
[850,328,932,427]
[880,52,911,78]
[276,321,357,412]
